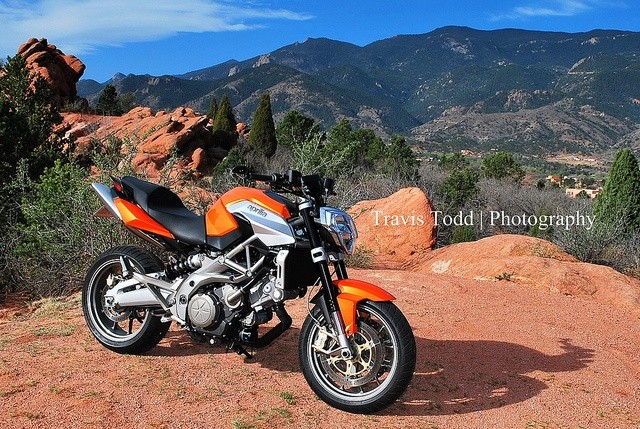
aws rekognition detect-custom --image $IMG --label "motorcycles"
[80,164,418,416]
